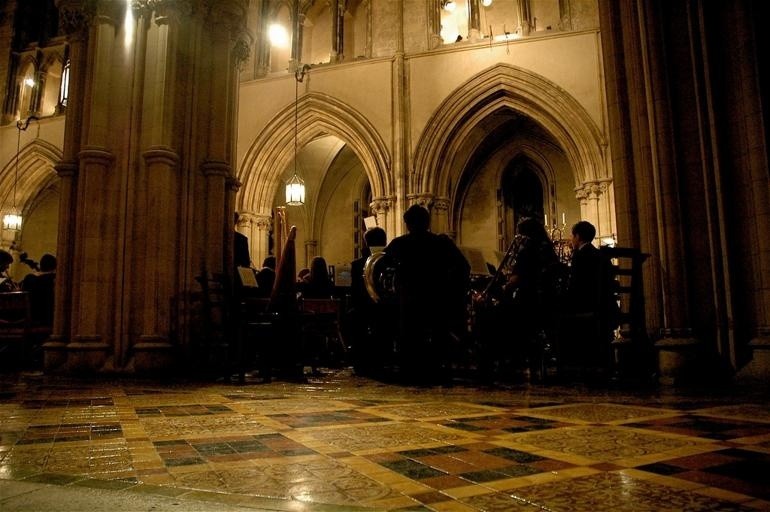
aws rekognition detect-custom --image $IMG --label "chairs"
[232,291,357,386]
[0,291,51,371]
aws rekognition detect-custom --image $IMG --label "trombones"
[552,228,562,262]
[475,234,521,310]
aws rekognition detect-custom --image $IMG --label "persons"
[232,201,624,387]
[0,249,17,292]
[23,249,56,328]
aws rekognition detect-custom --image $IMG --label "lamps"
[285,63,311,206]
[1,120,23,232]
